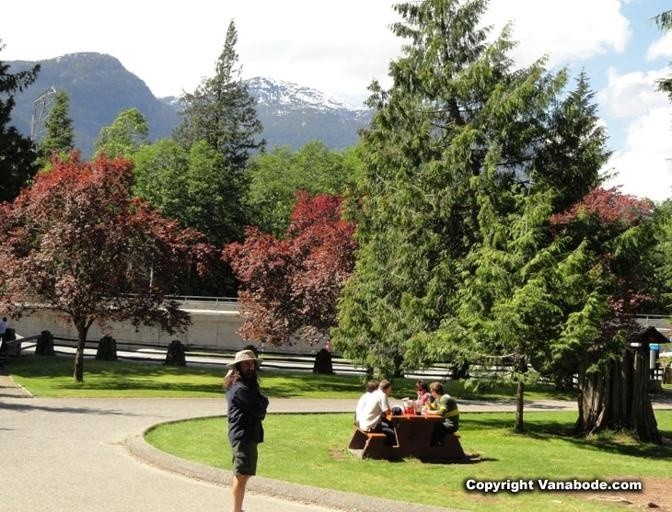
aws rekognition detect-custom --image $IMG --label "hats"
[226,350,263,369]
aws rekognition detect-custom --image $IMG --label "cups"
[402,397,423,415]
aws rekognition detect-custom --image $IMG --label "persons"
[0,317,8,349]
[222,350,269,512]
[402,380,431,411]
[423,382,459,447]
[359,379,395,446]
[356,380,379,426]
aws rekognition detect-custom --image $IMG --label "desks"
[384,412,444,457]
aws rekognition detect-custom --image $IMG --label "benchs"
[442,433,461,460]
[348,427,386,460]
